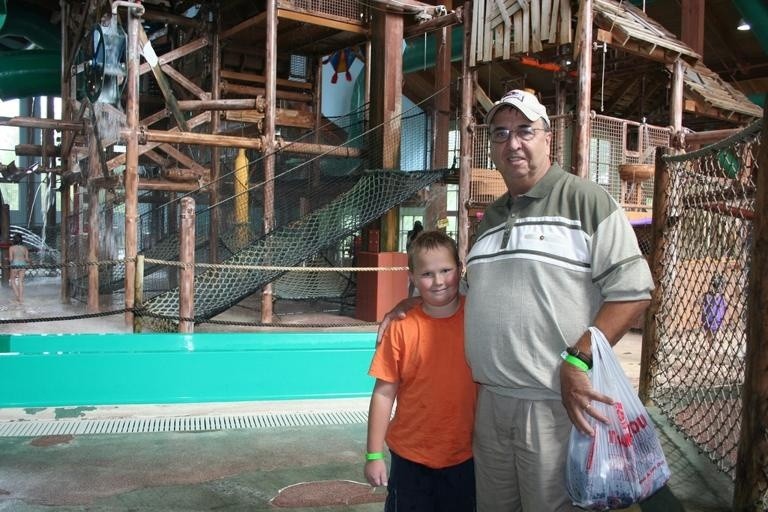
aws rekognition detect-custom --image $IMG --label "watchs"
[565,346,592,370]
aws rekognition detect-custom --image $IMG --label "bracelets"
[558,351,588,372]
[364,452,384,461]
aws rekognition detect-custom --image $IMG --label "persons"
[700,276,727,352]
[7,232,34,308]
[363,230,483,511]
[374,87,661,512]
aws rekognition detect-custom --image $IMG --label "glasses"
[489,126,546,144]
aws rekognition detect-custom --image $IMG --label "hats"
[484,89,551,129]
